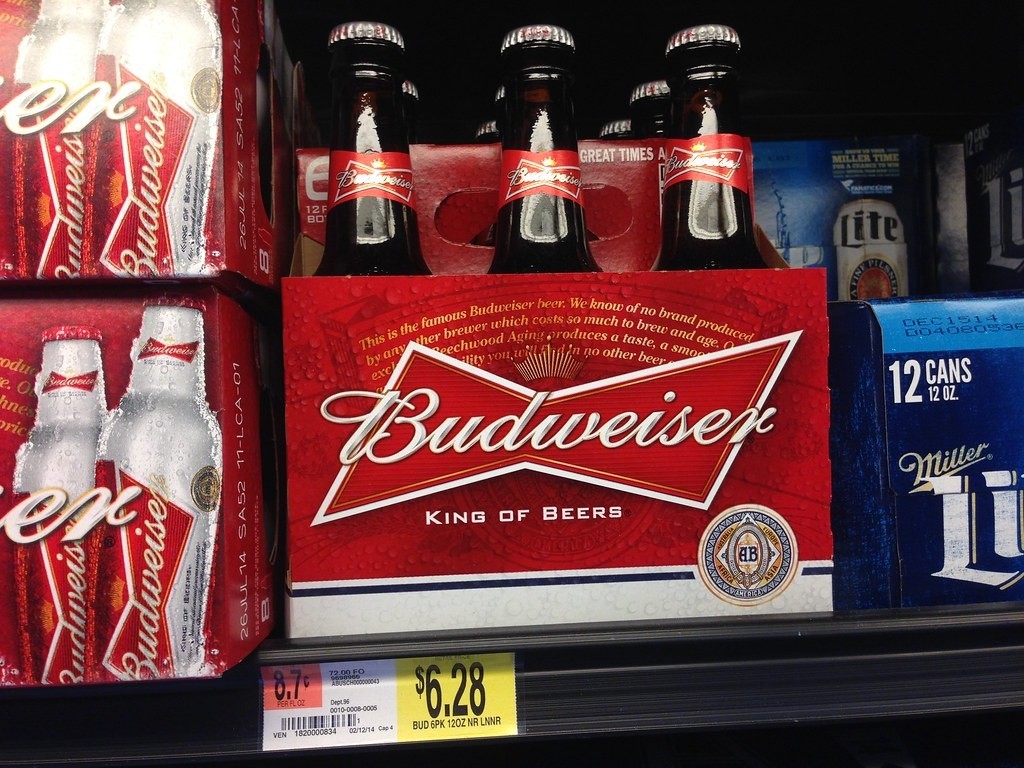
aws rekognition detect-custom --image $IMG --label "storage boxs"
[752,137,933,301]
[277,138,834,643]
[0,293,277,686]
[828,295,1024,615]
[0,0,303,295]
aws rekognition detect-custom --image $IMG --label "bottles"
[12,298,223,684]
[312,20,433,276]
[598,24,768,271]
[14,0,223,277]
[473,23,605,275]
